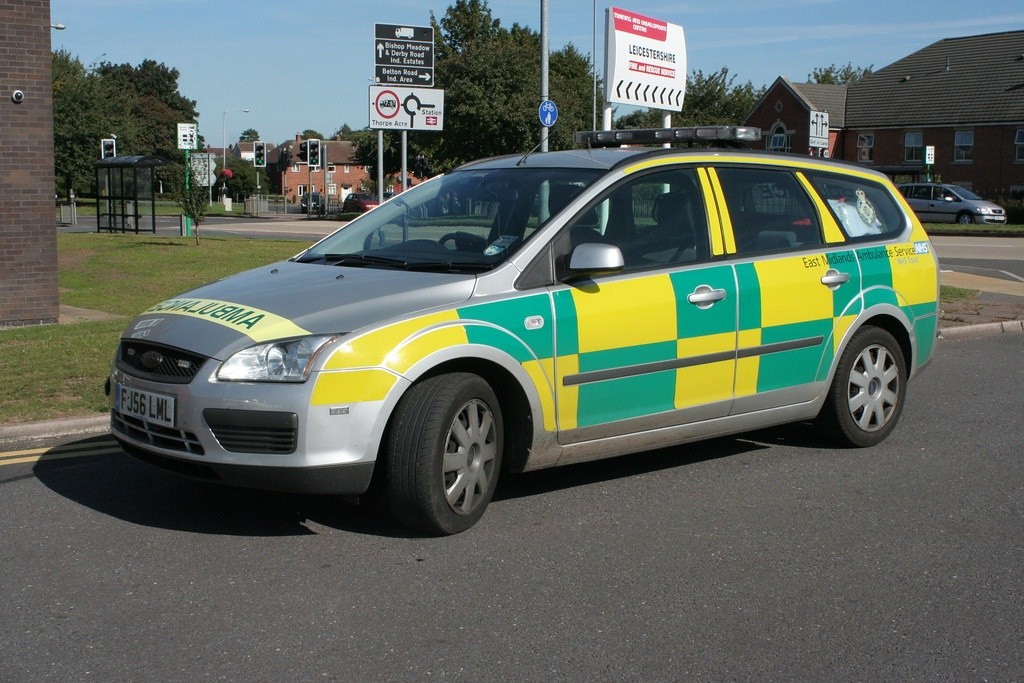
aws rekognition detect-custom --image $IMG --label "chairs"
[641,191,697,262]
[753,229,805,249]
[548,184,607,279]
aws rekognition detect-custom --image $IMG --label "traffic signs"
[374,23,434,88]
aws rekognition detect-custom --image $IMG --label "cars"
[342,193,378,212]
[112,124,942,536]
[896,181,1008,227]
[301,193,325,213]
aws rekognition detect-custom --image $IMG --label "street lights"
[222,109,250,198]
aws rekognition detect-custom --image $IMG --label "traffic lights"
[253,141,266,168]
[278,152,285,172]
[101,139,116,159]
[286,149,293,167]
[307,139,321,168]
[284,187,289,196]
[296,141,307,164]
